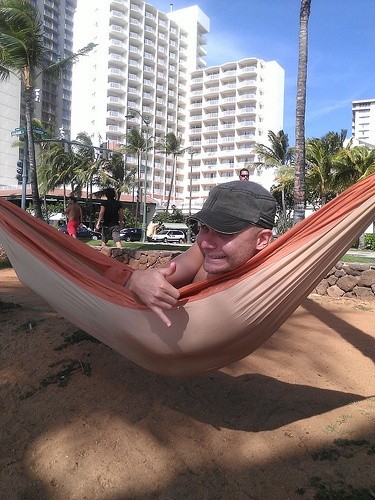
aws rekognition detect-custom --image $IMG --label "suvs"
[152,230,185,243]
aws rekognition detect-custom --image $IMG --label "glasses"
[240,175,249,177]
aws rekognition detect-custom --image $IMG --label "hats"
[185,181,276,234]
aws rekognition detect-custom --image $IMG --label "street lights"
[125,108,149,243]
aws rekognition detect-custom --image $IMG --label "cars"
[57,225,102,240]
[119,228,142,242]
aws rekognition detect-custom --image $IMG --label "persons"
[62,196,84,239]
[96,188,124,248]
[103,181,278,328]
[239,168,250,181]
